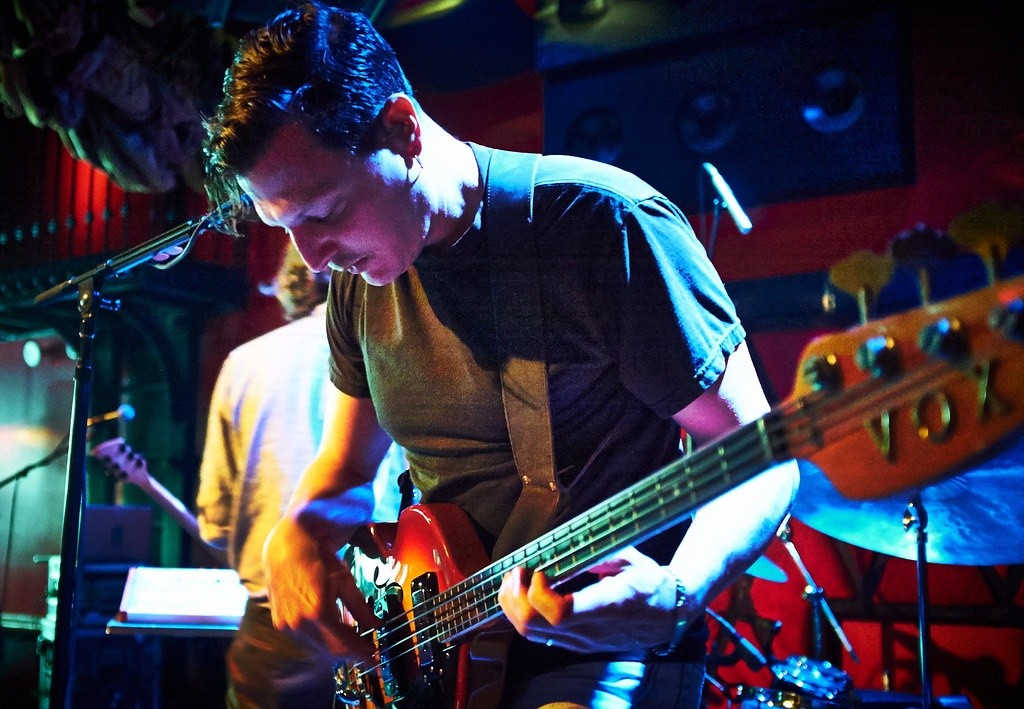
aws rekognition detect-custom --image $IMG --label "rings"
[546,636,555,646]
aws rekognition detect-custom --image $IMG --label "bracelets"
[654,565,689,656]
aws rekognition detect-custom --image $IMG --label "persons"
[203,0,802,709]
[197,245,421,709]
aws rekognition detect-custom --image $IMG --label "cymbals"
[789,427,1024,567]
[743,553,790,585]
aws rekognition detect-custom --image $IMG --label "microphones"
[85,404,136,427]
[702,162,753,234]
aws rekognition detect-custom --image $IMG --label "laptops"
[78,504,153,566]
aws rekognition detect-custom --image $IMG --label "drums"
[739,652,970,708]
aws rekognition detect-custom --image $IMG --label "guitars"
[332,238,1024,709]
[89,437,199,540]
